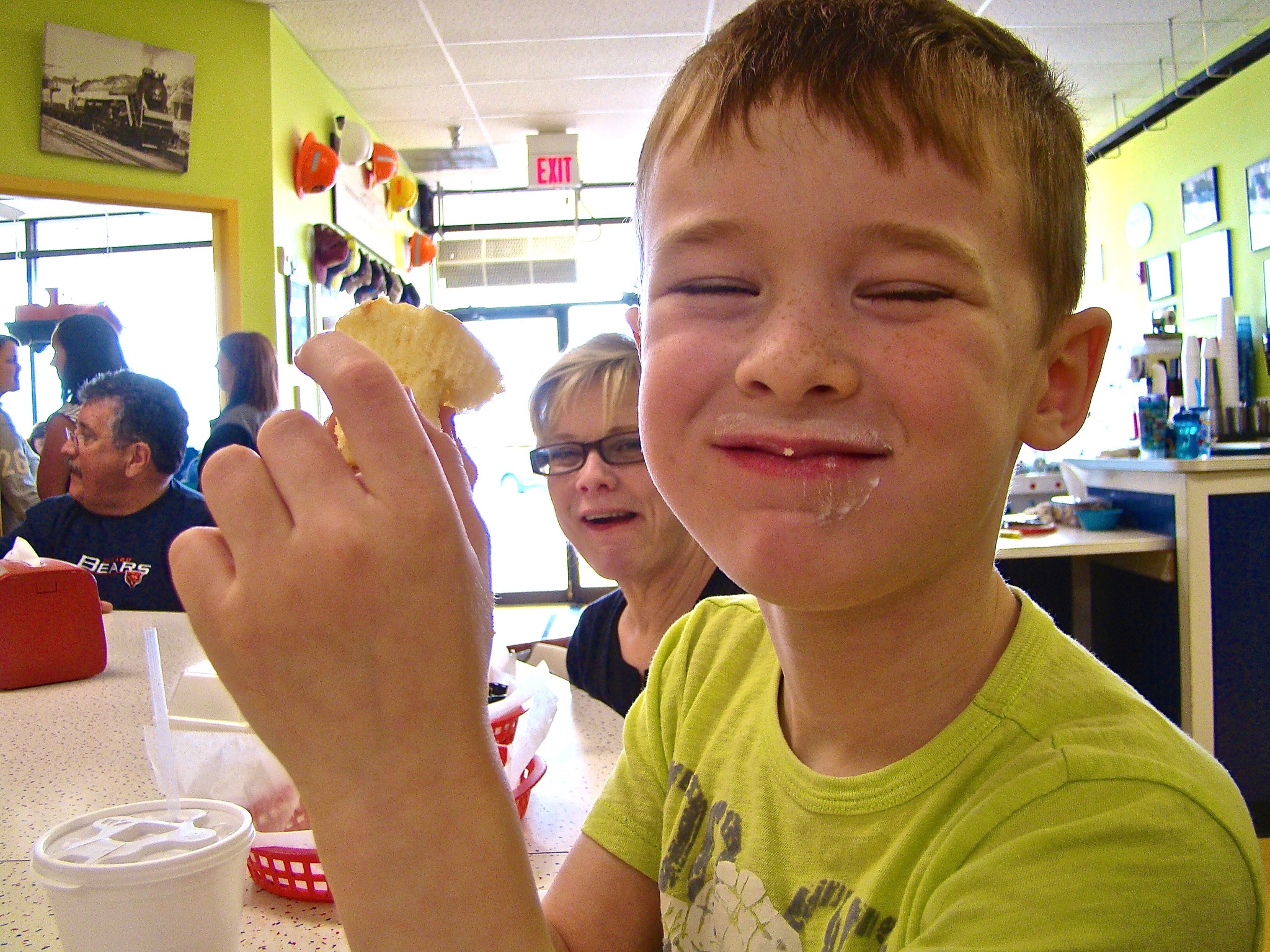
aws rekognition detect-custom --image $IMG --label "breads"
[327,295,502,468]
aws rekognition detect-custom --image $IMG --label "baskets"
[245,754,551,902]
[484,701,528,763]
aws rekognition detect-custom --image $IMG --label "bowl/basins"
[1051,495,1099,529]
[1075,509,1123,532]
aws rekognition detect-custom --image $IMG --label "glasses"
[529,430,647,476]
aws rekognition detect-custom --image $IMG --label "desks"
[0,604,624,952]
[993,453,1270,841]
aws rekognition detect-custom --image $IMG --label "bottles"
[1173,406,1202,460]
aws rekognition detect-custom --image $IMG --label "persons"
[37,315,128,499]
[26,421,46,456]
[0,370,221,613]
[0,335,41,537]
[166,1,1270,952]
[196,332,280,493]
[528,335,749,716]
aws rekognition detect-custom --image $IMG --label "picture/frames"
[1145,156,1270,333]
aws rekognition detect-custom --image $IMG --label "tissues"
[0,535,108,692]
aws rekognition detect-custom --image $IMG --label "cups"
[1138,392,1168,460]
[1180,297,1256,438]
[1249,405,1270,437]
[28,797,257,952]
[1222,407,1249,442]
[1189,407,1212,460]
